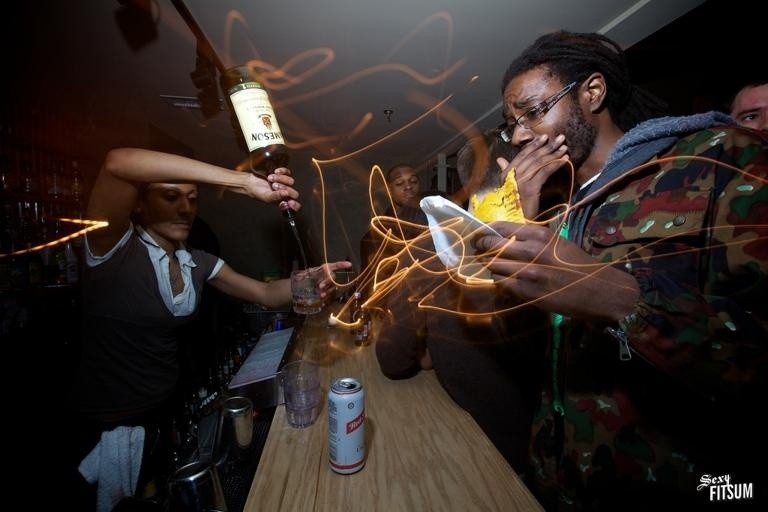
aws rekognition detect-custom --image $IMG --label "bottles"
[1,151,83,286]
[219,66,295,223]
[350,292,372,347]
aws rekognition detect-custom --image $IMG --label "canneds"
[328,378,367,474]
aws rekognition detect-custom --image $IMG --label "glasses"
[500,82,577,143]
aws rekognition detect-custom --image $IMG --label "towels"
[78,425,145,512]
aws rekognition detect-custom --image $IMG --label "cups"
[291,268,322,314]
[282,360,321,427]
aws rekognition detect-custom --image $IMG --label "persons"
[77,127,353,512]
[376,128,561,472]
[466,30,768,512]
[358,164,449,318]
[721,61,768,135]
